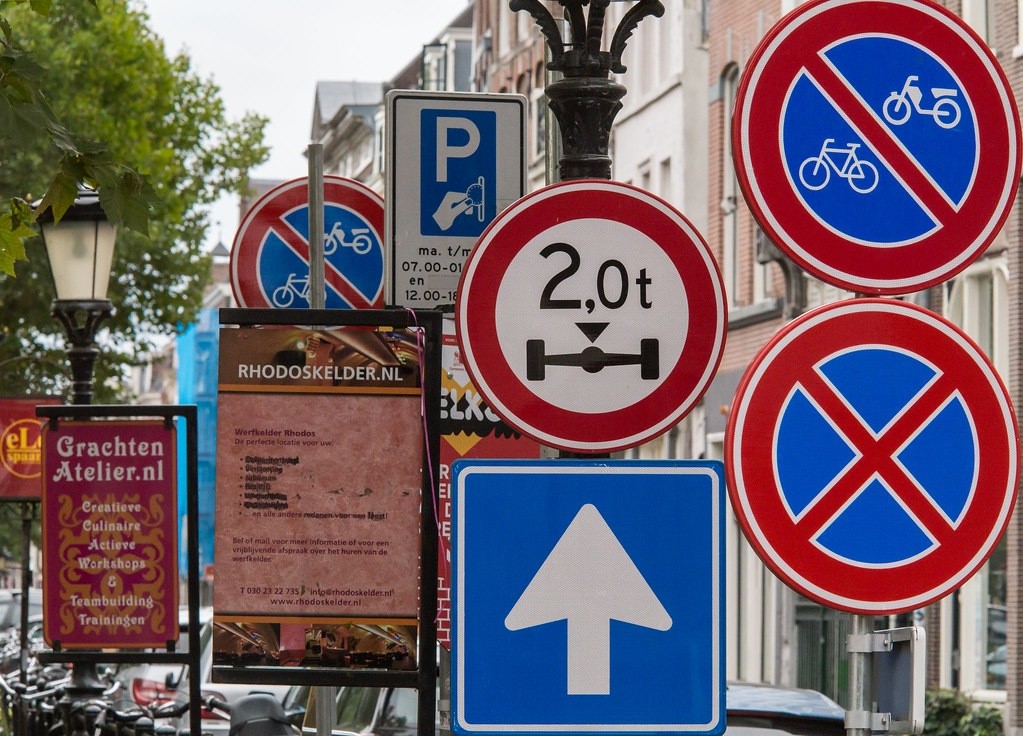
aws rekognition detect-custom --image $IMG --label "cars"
[1,585,450,736]
[723,683,846,736]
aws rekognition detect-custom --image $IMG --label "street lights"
[27,176,121,701]
